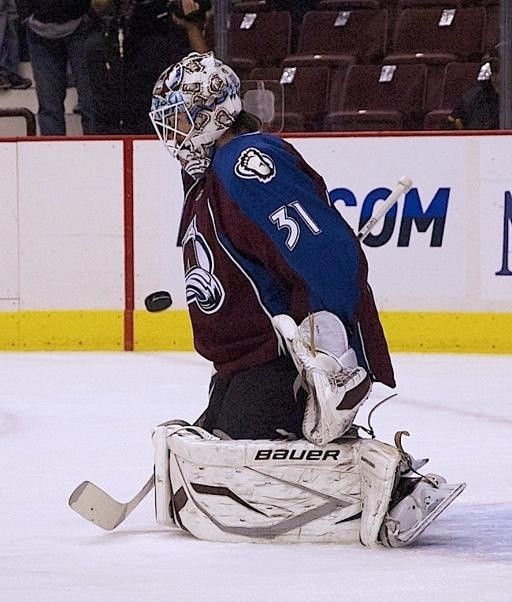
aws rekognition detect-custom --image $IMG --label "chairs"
[226,0,512,133]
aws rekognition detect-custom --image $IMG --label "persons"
[91,0,212,133]
[11,0,110,136]
[147,52,479,549]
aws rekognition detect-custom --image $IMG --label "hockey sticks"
[68,175,413,530]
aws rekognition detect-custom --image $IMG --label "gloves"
[269,310,374,445]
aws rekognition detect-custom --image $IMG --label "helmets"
[148,50,244,181]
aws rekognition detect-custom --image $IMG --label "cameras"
[165,0,212,23]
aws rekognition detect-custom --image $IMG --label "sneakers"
[389,472,446,534]
